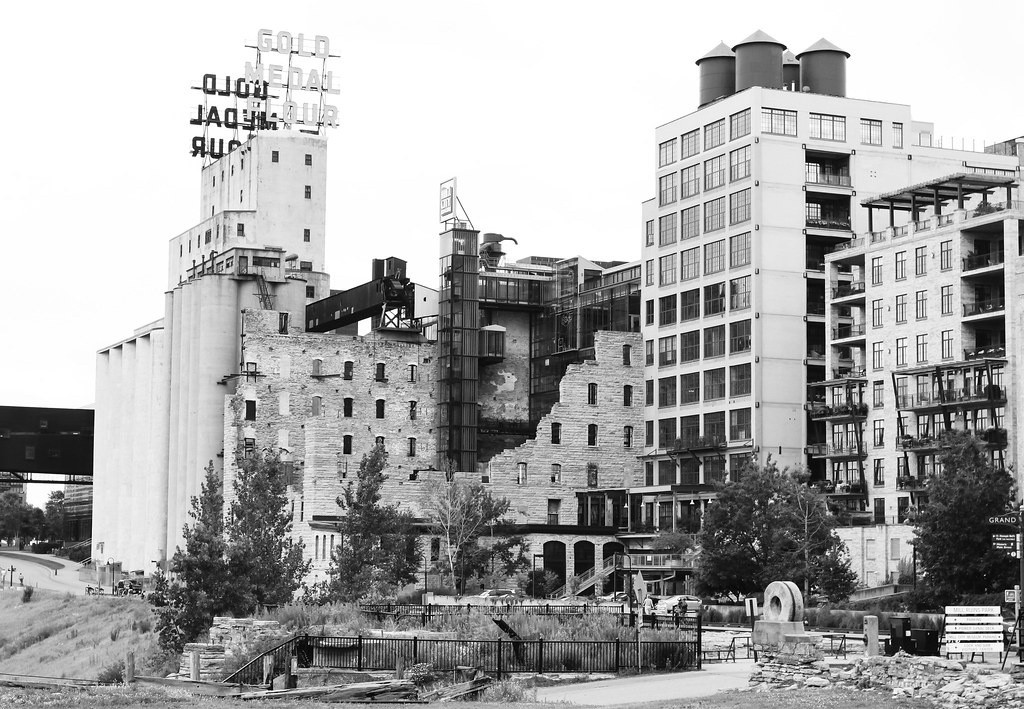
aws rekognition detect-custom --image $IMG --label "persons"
[85,581,134,598]
[643,595,654,614]
[674,597,687,616]
[19,573,24,584]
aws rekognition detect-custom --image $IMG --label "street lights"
[532,554,544,601]
[489,518,497,574]
[107,557,114,595]
[614,552,624,602]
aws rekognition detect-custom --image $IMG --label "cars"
[612,594,629,603]
[658,594,702,613]
[631,595,660,610]
[597,591,623,602]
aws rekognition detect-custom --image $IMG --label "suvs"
[467,589,515,599]
